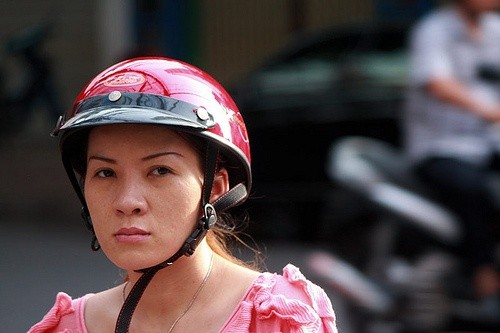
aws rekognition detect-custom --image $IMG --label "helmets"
[52,57,251,208]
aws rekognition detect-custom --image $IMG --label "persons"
[25,58,338,333]
[404,0,500,281]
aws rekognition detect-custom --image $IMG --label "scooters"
[306,64,500,333]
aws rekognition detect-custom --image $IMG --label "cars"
[213,23,409,243]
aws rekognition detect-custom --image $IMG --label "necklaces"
[123,252,214,333]
